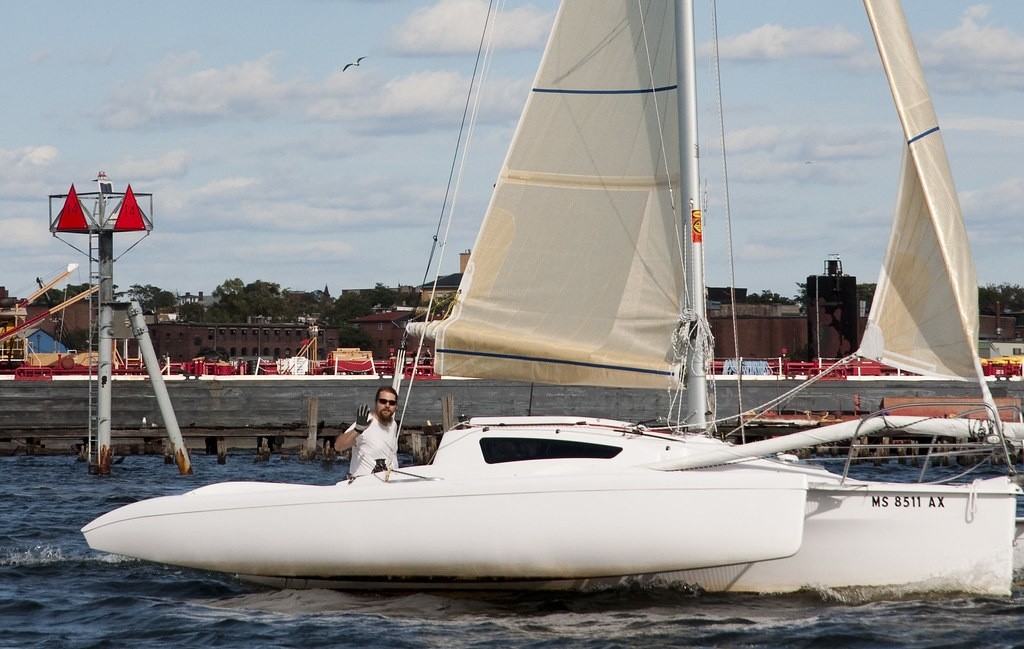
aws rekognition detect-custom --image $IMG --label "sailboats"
[77,0,1024,607]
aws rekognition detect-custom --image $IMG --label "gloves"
[354,404,373,434]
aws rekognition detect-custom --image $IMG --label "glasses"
[378,399,396,406]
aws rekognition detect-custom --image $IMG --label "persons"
[333,385,399,477]
